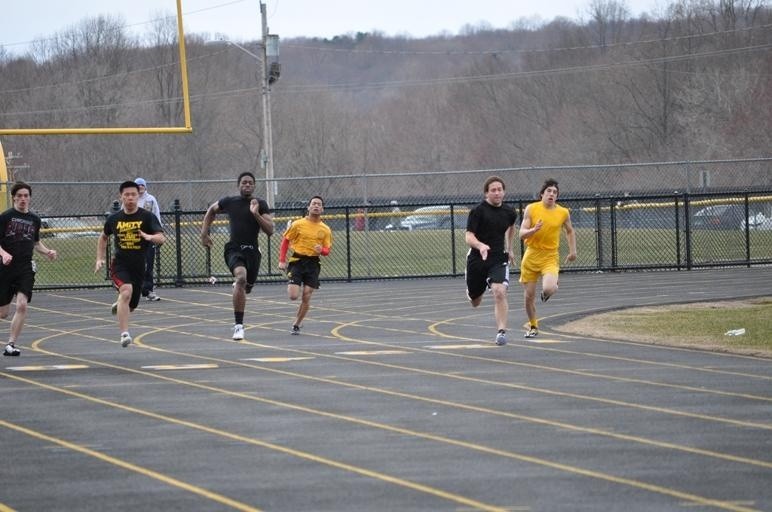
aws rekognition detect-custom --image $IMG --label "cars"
[694,204,754,229]
[40,218,102,239]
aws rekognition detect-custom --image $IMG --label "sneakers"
[121,334,132,347]
[495,329,506,346]
[290,326,300,336]
[233,323,244,340]
[3,342,20,356]
[523,283,558,338]
[145,292,161,301]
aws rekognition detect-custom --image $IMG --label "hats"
[134,177,147,188]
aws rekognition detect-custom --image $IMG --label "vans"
[386,206,469,230]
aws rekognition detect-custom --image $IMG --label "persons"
[278,195,333,336]
[92,180,167,347]
[356,208,366,229]
[131,178,163,302]
[2,182,58,357]
[517,179,577,341]
[198,171,275,340]
[463,176,517,346]
[391,199,401,229]
